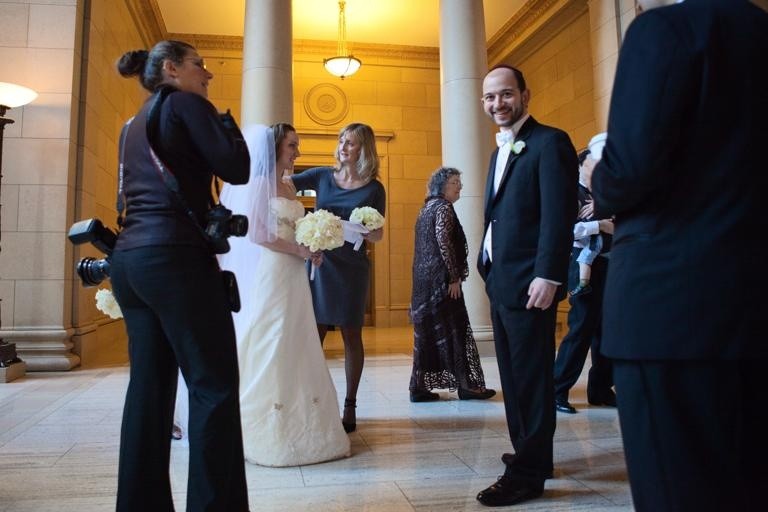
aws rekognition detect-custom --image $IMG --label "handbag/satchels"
[222,272,241,313]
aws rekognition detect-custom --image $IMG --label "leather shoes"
[458,387,496,400]
[502,453,554,479]
[555,398,575,413]
[342,398,356,432]
[411,390,440,402]
[477,472,544,506]
[589,395,617,407]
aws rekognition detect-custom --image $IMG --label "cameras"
[67,218,117,288]
[203,203,249,255]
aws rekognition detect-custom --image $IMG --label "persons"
[103,41,253,512]
[475,64,580,507]
[554,148,617,413]
[214,121,352,469]
[568,232,604,297]
[579,0,768,512]
[282,123,386,434]
[409,166,497,402]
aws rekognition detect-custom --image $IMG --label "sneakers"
[569,284,593,297]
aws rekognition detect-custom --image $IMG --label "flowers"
[349,205,386,236]
[510,139,526,155]
[93,288,121,323]
[295,208,346,256]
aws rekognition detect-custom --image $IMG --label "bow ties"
[495,129,515,147]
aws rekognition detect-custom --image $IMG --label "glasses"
[182,55,207,71]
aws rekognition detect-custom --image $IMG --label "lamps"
[322,1,362,79]
[0,79,40,252]
